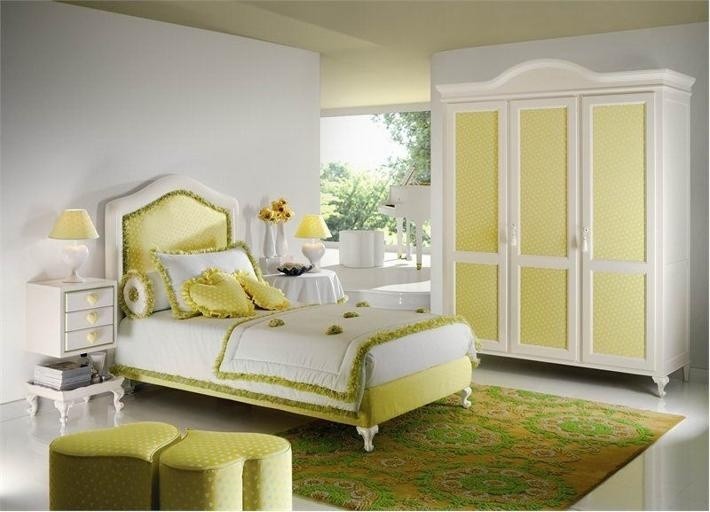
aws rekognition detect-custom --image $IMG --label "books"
[32,361,90,391]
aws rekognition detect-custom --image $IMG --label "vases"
[264,222,276,258]
[276,223,288,257]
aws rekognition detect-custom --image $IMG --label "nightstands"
[27,276,118,359]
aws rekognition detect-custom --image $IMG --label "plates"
[277,265,313,276]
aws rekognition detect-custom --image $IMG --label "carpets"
[271,380,685,511]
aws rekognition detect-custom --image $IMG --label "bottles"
[78,353,90,370]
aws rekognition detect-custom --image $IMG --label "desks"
[26,374,125,437]
[265,269,345,306]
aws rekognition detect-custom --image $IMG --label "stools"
[161,428,292,511]
[340,230,385,267]
[50,422,179,511]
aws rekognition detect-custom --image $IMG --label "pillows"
[235,268,288,310]
[151,240,262,320]
[120,270,171,318]
[184,267,254,316]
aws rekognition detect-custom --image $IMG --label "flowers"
[259,209,276,221]
[272,200,292,223]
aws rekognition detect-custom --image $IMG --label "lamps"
[48,209,99,283]
[294,215,332,272]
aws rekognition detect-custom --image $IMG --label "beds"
[105,174,478,452]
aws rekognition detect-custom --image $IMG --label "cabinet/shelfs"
[436,59,697,398]
